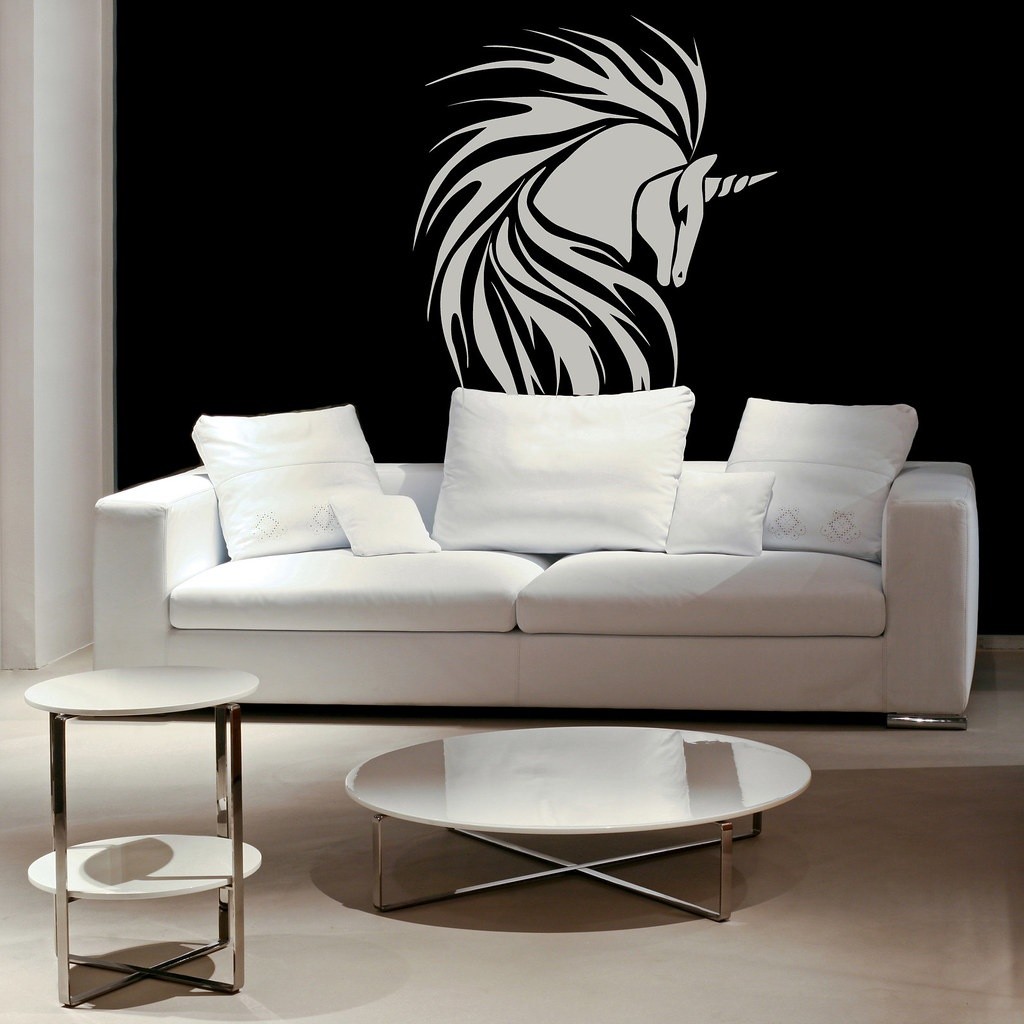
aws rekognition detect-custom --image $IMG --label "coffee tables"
[346,725,812,922]
[24,664,264,1007]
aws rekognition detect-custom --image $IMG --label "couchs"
[93,461,978,732]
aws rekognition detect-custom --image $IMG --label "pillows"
[666,473,774,557]
[726,398,919,563]
[326,490,441,556]
[430,386,695,553]
[192,404,385,563]
